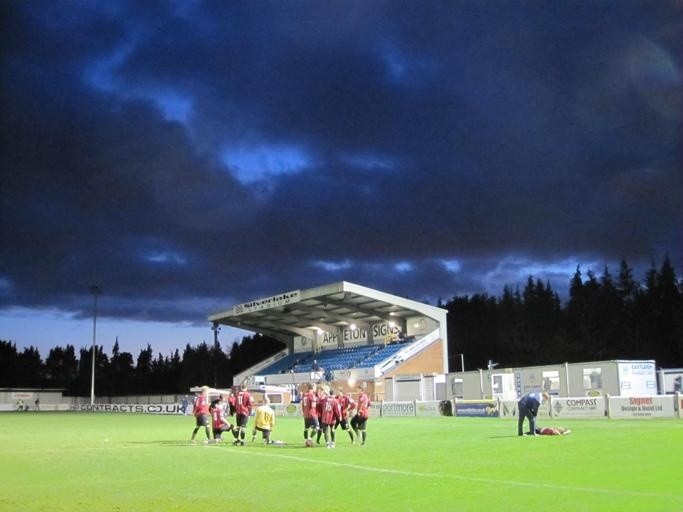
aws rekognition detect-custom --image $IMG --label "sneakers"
[188,438,365,449]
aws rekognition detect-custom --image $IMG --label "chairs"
[254,342,411,375]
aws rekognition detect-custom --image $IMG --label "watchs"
[16,397,24,414]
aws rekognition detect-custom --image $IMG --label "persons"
[35,398,40,412]
[517,391,550,436]
[179,397,188,415]
[524,427,572,436]
[386,327,405,345]
[189,382,372,449]
[310,359,319,371]
